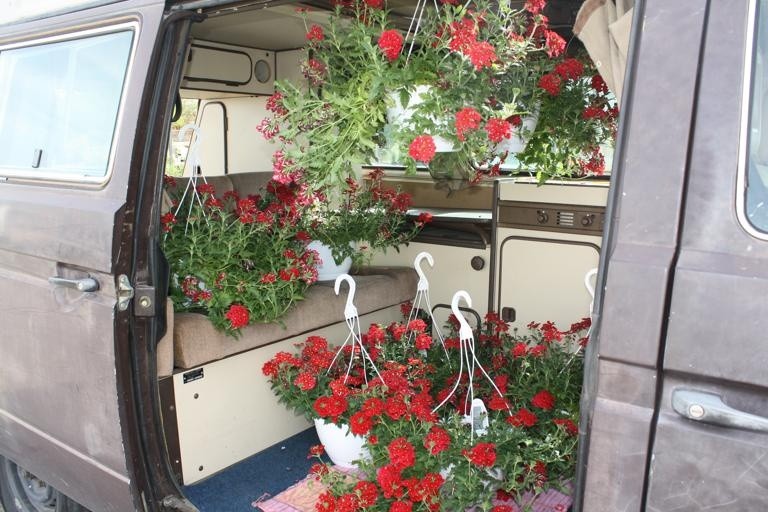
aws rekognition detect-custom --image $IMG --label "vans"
[1,1,766,512]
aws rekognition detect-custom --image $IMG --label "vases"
[310,415,375,471]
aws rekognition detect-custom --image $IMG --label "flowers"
[264,4,620,180]
[172,163,430,330]
[261,336,423,420]
[378,285,599,512]
